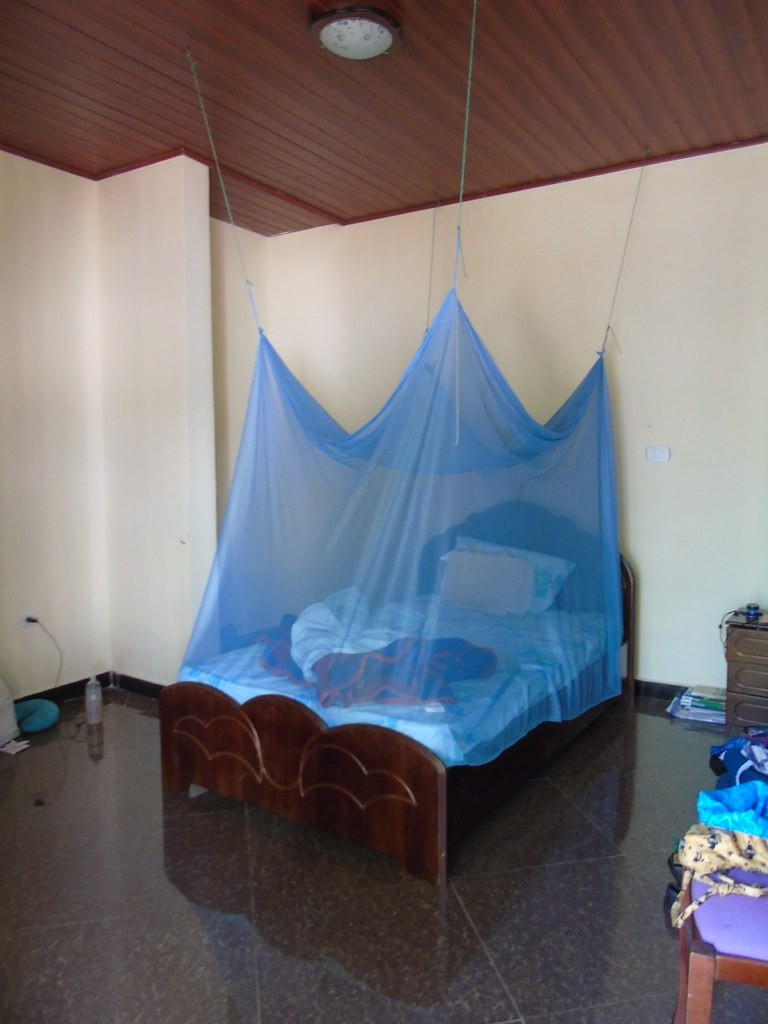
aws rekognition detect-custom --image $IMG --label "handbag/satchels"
[670,824,768,928]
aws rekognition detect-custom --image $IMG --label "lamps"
[309,5,400,60]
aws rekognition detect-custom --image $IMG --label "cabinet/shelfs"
[724,607,768,738]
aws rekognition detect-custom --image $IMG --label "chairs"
[674,867,768,1024]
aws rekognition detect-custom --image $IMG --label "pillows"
[442,550,537,615]
[15,699,59,732]
[455,532,576,614]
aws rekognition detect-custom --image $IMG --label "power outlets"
[20,613,34,625]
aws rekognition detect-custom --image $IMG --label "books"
[682,687,726,716]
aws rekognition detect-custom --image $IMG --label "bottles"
[85,672,102,724]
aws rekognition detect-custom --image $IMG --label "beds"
[160,497,637,888]
